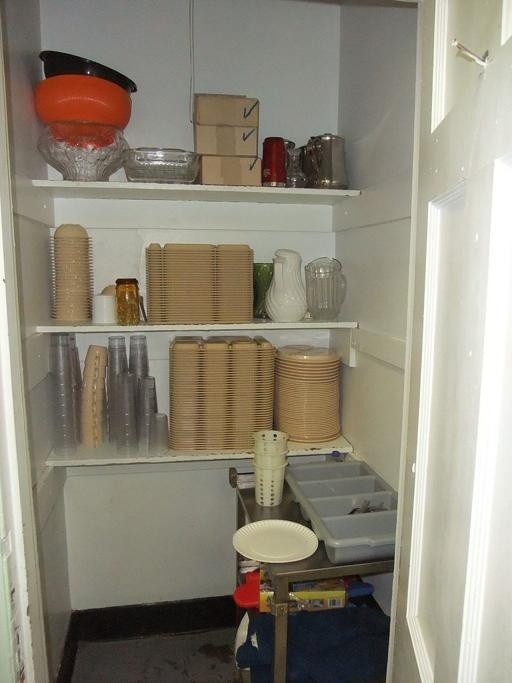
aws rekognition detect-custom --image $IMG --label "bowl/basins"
[34,73,133,149]
[48,223,92,323]
[37,48,137,98]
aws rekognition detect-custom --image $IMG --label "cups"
[304,253,349,320]
[48,332,168,457]
[254,260,273,320]
[92,295,118,325]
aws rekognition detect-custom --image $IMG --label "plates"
[231,519,319,564]
[274,343,344,444]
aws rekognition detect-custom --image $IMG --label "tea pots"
[264,245,308,323]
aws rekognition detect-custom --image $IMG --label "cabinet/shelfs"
[227,475,395,683]
[29,178,359,472]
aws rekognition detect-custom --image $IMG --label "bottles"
[115,278,140,326]
[262,136,287,187]
